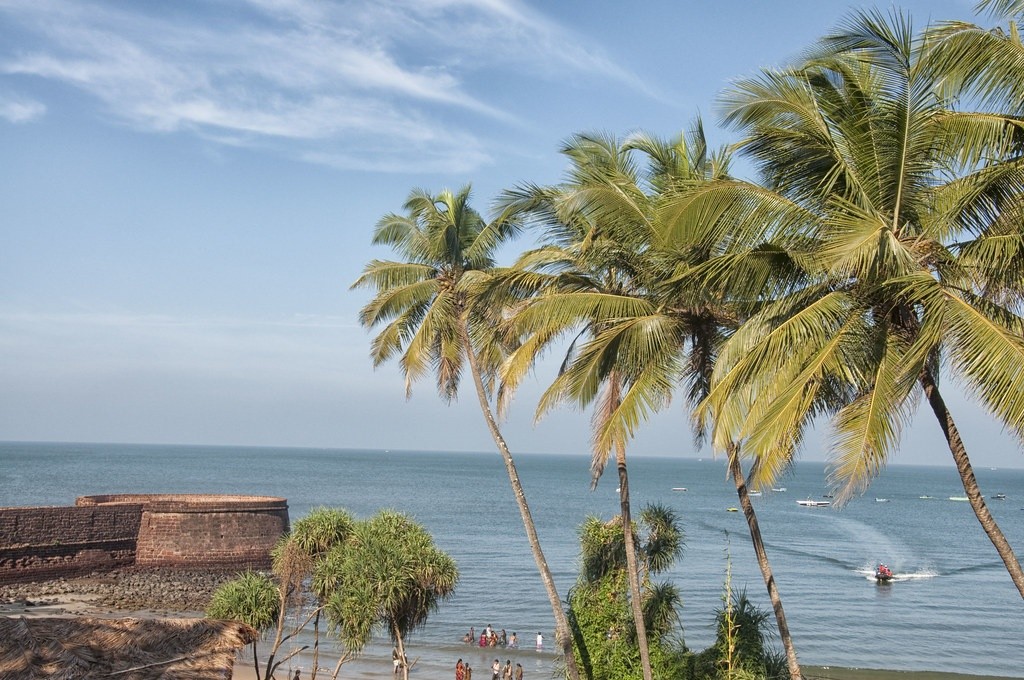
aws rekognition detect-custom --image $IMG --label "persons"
[479,624,506,647]
[393,645,408,674]
[491,659,523,680]
[464,627,474,643]
[456,659,472,680]
[880,563,892,577]
[536,632,544,648]
[293,670,300,680]
[508,632,517,647]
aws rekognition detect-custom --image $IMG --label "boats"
[796,500,831,508]
[671,488,687,492]
[919,495,933,499]
[991,494,1005,500]
[748,490,762,497]
[728,508,738,512]
[873,497,889,504]
[875,573,893,581]
[949,496,971,501]
[773,488,786,493]
